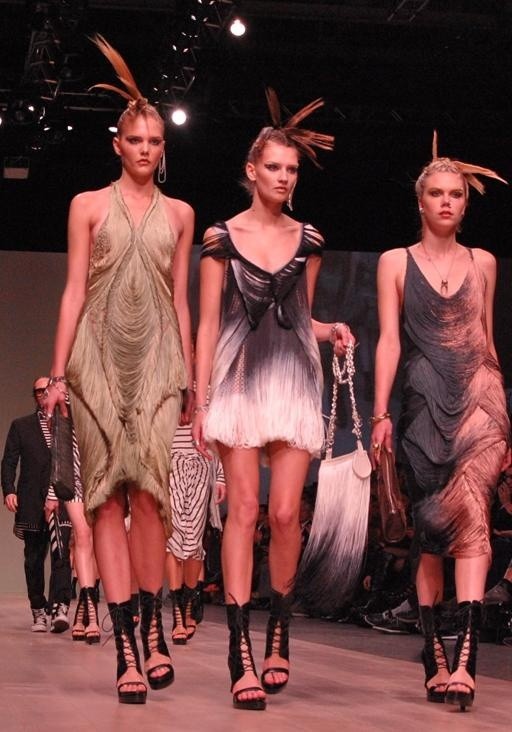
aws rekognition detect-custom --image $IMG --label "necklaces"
[416,239,462,295]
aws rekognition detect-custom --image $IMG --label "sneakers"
[51,601,69,633]
[31,608,47,631]
[206,571,271,610]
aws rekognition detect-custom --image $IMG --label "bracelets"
[328,321,347,345]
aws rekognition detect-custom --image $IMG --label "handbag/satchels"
[379,448,407,543]
[301,450,372,606]
[48,511,65,561]
[51,403,75,500]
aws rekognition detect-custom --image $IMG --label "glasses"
[34,388,45,397]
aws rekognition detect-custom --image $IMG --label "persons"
[44,367,111,644]
[43,98,199,708]
[185,125,361,714]
[160,377,227,646]
[2,365,81,635]
[192,444,508,645]
[368,157,509,711]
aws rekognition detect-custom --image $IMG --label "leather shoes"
[292,585,420,633]
[483,579,511,604]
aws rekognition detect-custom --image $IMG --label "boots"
[171,581,205,644]
[138,586,174,689]
[446,600,482,712]
[108,601,146,704]
[261,589,294,694]
[228,604,266,710]
[419,603,450,703]
[74,578,100,643]
